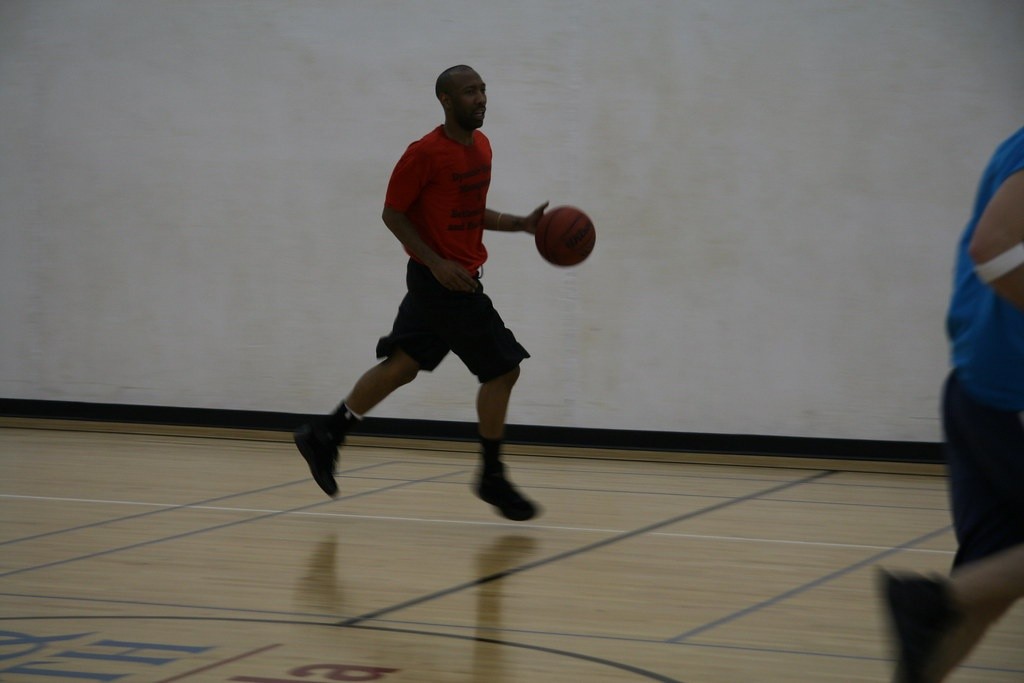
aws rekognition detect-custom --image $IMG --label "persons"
[292,64,552,521]
[879,126,1024,683]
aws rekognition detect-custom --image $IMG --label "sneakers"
[474,474,535,521]
[295,416,345,496]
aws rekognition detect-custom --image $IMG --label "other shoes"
[877,571,962,683]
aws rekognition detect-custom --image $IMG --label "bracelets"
[496,213,503,230]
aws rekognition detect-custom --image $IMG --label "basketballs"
[534,206,596,266]
